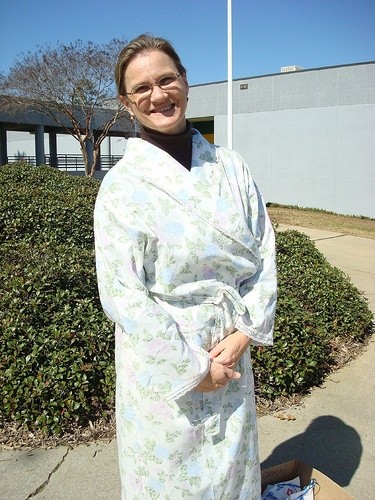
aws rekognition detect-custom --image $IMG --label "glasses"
[125,74,186,95]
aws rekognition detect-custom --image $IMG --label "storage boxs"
[261,460,355,500]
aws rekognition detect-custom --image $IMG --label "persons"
[93,34,277,500]
